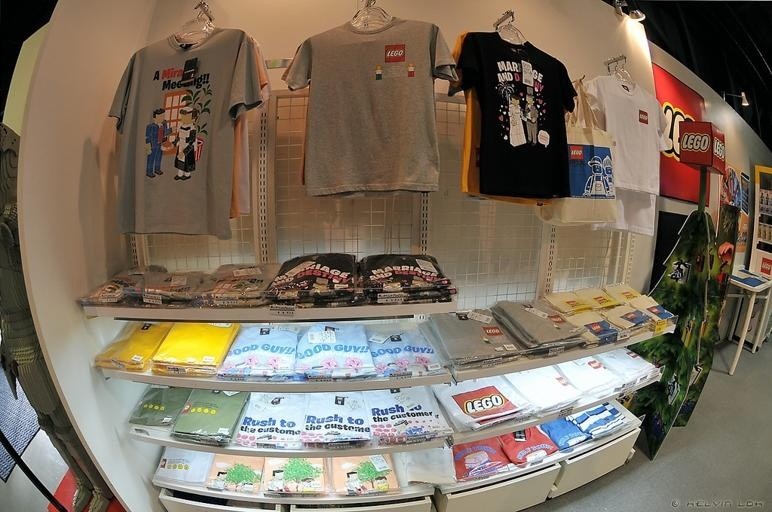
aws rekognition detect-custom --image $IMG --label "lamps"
[720,90,752,107]
[614,0,647,21]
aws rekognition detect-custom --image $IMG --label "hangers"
[171,0,215,47]
[606,53,635,87]
[496,10,526,46]
[352,0,391,30]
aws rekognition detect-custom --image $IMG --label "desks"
[720,269,772,376]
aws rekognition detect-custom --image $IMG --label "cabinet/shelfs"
[736,165,772,350]
[82,259,674,512]
